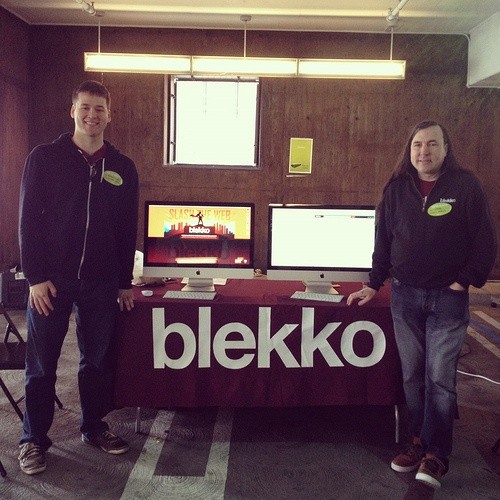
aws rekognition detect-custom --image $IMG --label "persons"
[19,80,139,475]
[347,122,496,489]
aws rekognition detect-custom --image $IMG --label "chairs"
[0,303,65,426]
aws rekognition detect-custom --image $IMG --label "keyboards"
[162,289,216,300]
[290,291,344,304]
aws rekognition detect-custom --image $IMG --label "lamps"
[83,0,406,78]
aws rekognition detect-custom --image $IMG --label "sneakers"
[415,457,445,490]
[18,442,46,474]
[391,443,426,473]
[82,431,130,454]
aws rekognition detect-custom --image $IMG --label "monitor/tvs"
[141,200,255,292]
[266,203,376,294]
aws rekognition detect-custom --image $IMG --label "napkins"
[181,277,228,285]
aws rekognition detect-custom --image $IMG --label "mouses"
[141,289,153,297]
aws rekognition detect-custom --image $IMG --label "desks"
[108,276,406,442]
